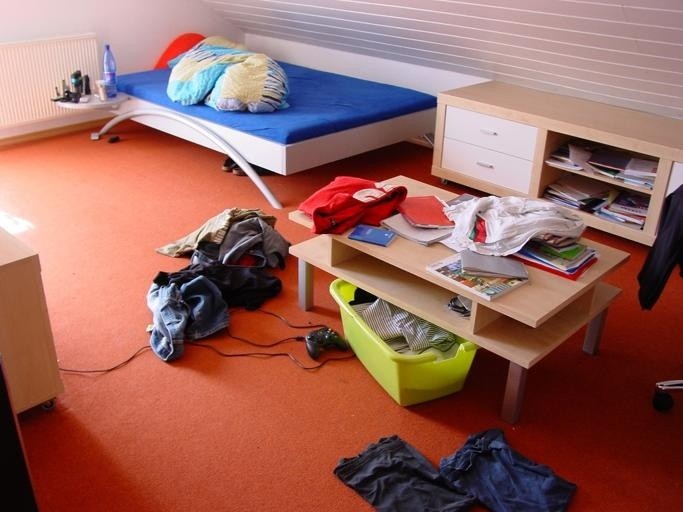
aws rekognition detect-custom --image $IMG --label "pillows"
[154,33,205,70]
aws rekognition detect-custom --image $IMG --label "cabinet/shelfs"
[1,228,67,415]
[430,79,683,248]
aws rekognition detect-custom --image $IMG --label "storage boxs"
[327,278,477,410]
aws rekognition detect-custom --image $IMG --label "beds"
[53,32,436,210]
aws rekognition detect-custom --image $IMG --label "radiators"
[0,31,102,131]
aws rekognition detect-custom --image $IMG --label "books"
[462,254,529,280]
[380,213,459,247]
[347,224,398,248]
[394,194,458,228]
[426,248,528,302]
[445,192,476,207]
[506,233,599,282]
[542,139,658,231]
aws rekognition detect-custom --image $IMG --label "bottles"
[96,80,108,101]
[101,45,119,99]
[70,70,91,97]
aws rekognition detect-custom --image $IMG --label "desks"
[288,174,630,426]
